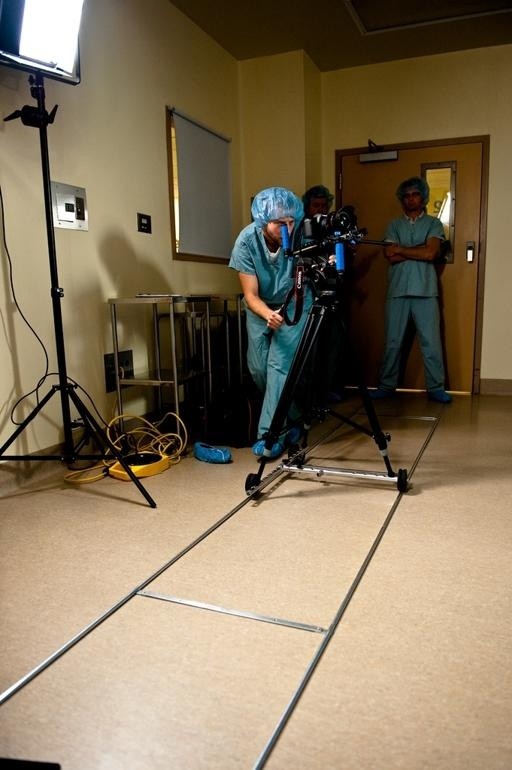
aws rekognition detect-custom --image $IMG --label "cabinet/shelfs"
[105,294,244,459]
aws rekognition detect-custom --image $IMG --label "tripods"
[245,277,407,498]
[0,72,157,510]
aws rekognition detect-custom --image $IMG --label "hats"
[251,186,304,231]
[395,177,430,203]
[303,185,335,215]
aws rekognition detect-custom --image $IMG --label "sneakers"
[370,389,395,398]
[288,428,299,444]
[252,440,282,458]
[194,442,232,463]
[429,390,451,404]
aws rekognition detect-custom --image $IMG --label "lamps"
[0,0,161,512]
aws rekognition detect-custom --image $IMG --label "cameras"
[277,204,368,277]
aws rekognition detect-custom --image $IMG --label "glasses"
[404,192,420,198]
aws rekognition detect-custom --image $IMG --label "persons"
[303,185,334,218]
[368,176,452,403]
[228,186,313,457]
